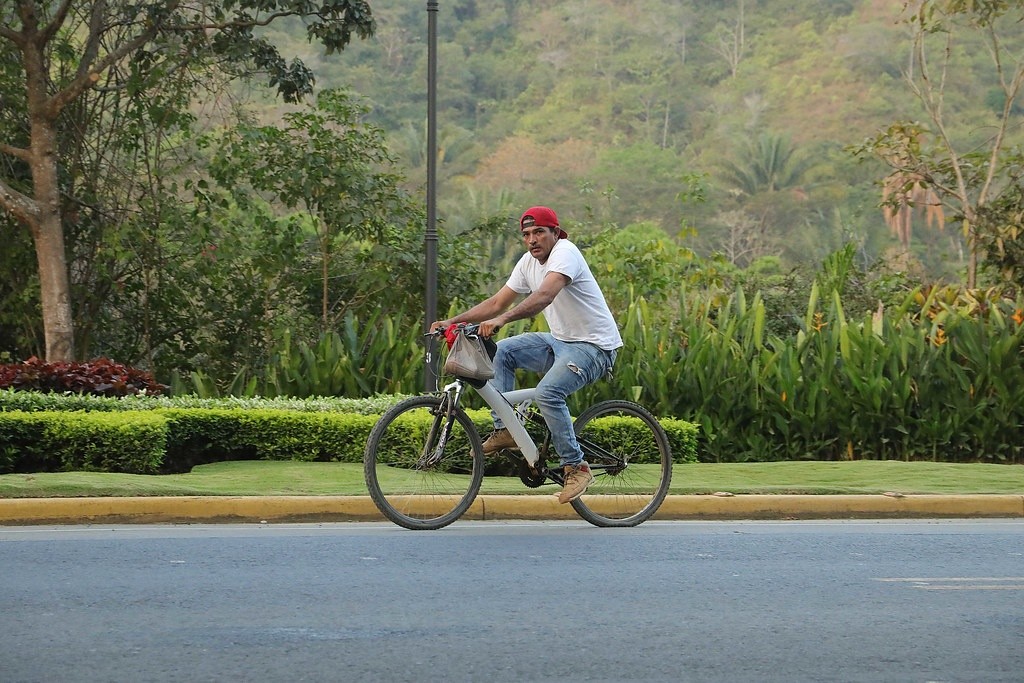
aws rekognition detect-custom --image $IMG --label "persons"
[428,207,623,505]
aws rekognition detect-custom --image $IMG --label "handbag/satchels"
[443,322,497,389]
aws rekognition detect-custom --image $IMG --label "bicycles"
[363,321,675,531]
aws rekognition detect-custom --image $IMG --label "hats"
[520,207,567,239]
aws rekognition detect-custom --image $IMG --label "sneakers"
[470,429,519,455]
[559,460,595,504]
